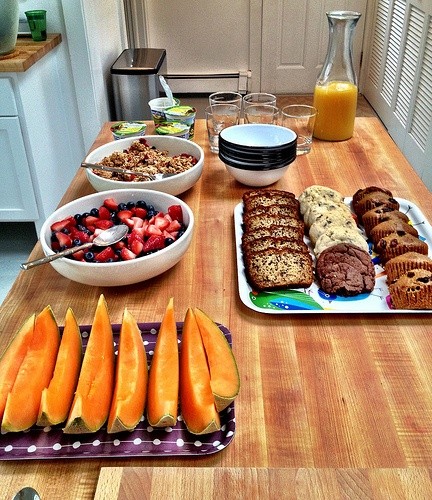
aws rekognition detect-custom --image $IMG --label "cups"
[205,91,242,155]
[281,104,317,155]
[24,10,47,41]
[243,93,279,125]
[110,121,147,140]
[148,96,180,125]
[155,122,190,140]
[163,105,197,141]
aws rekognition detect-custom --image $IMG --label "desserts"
[353,187,432,311]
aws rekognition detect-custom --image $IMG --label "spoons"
[81,162,177,182]
[19,225,129,271]
[13,487,41,500]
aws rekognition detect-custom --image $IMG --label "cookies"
[297,185,376,297]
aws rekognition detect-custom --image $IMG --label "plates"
[0,323,236,463]
[234,197,432,314]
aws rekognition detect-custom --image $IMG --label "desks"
[0,93,432,500]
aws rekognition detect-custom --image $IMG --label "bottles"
[308,10,362,141]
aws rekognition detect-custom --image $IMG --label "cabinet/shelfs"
[0,40,80,241]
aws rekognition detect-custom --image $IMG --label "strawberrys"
[51,198,183,261]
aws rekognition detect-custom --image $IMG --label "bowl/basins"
[40,188,194,286]
[218,124,298,187]
[85,133,204,196]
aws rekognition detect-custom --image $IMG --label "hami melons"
[0,294,240,435]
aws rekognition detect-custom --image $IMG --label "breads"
[241,189,314,290]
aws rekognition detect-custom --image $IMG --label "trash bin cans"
[110,48,168,121]
